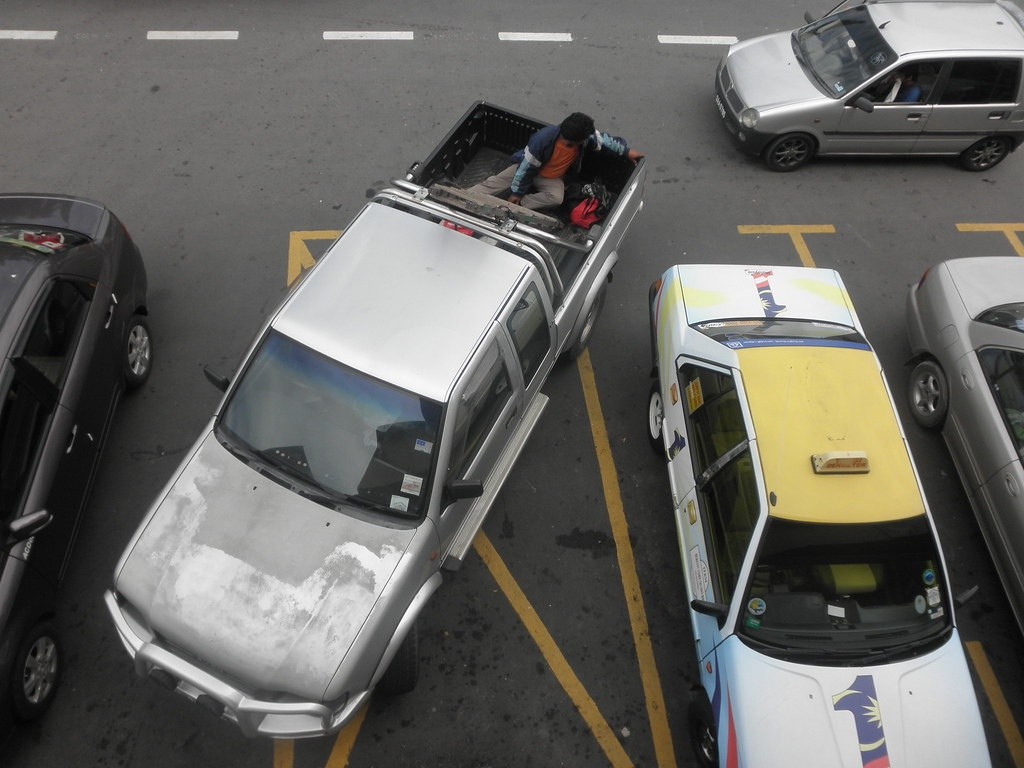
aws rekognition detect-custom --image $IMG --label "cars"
[904,254,1024,637]
[643,261,1000,768]
[0,192,159,730]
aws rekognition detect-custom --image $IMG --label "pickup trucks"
[97,95,649,742]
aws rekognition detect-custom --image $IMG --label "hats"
[561,112,595,141]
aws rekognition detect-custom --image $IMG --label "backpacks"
[562,182,614,230]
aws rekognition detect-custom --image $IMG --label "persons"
[466,111,644,210]
[875,64,920,103]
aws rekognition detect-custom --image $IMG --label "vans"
[713,0,1024,172]
